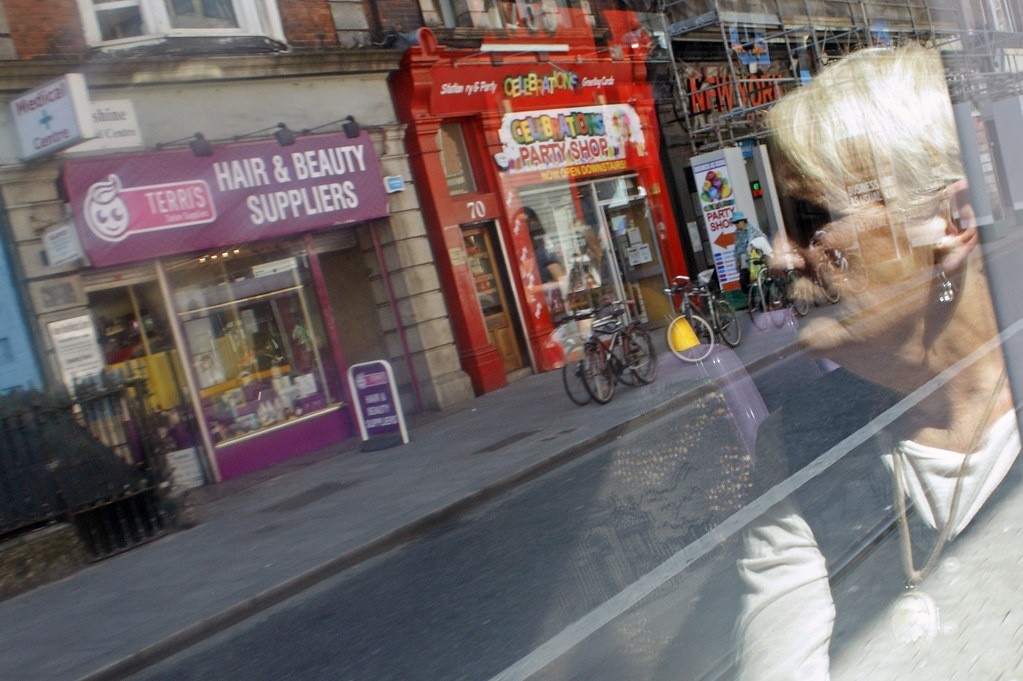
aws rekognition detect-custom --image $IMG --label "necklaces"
[890,367,1007,594]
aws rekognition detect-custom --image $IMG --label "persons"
[731,211,768,314]
[512,206,564,295]
[733,47,1022,679]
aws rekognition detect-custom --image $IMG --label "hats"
[731,211,746,222]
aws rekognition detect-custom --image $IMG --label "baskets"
[552,321,583,356]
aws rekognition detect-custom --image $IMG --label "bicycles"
[662,270,742,364]
[555,300,657,407]
[745,232,871,332]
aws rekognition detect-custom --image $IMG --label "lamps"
[301,115,360,139]
[157,132,214,157]
[232,122,296,147]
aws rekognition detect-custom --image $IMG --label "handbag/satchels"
[750,248,766,281]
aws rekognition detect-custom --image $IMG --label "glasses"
[734,220,742,224]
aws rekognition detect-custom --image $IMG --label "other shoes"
[749,305,757,313]
